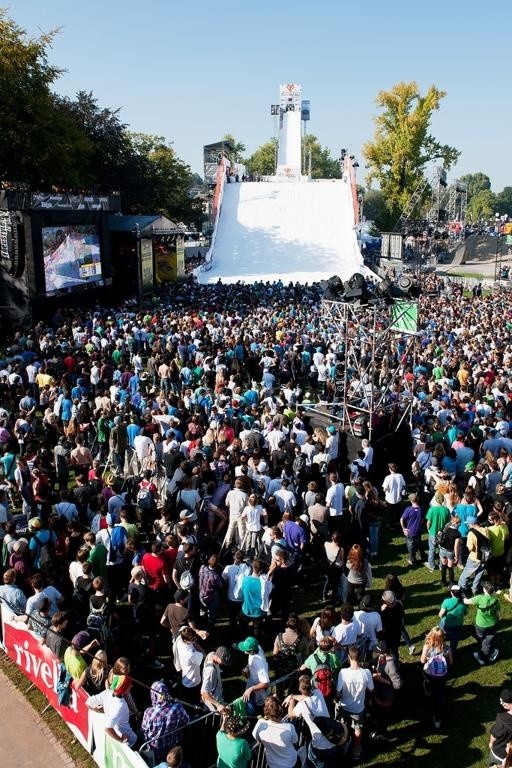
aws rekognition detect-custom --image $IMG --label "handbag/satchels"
[309,710,349,746]
[411,461,421,477]
[435,530,446,544]
[470,528,493,563]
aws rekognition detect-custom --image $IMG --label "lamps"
[320,270,423,303]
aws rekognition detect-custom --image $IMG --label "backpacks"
[474,476,485,497]
[34,531,57,572]
[423,646,448,678]
[310,654,336,699]
[87,597,112,644]
[179,566,194,592]
[274,634,299,678]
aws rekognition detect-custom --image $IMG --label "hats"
[372,640,389,653]
[224,715,250,737]
[71,631,90,648]
[111,674,131,694]
[179,510,194,518]
[13,537,28,553]
[463,517,478,524]
[216,637,258,667]
[467,461,474,468]
[299,514,309,526]
[359,594,384,610]
[113,416,121,424]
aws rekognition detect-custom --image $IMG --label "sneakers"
[489,649,499,662]
[149,659,165,669]
[408,646,416,655]
[474,652,485,665]
[424,562,435,571]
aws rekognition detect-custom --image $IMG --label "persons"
[2,210,511,768]
[228,171,264,182]
